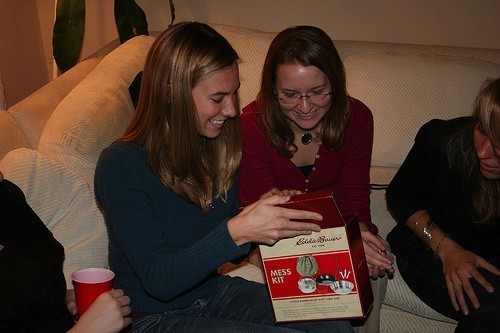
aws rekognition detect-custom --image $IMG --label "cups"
[70,267,116,319]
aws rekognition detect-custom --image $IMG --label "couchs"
[0,22,500,333]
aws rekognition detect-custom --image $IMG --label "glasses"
[275,89,332,104]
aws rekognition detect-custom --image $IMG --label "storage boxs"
[238,188,374,323]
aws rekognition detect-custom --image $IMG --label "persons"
[386,77,500,333]
[240,25,395,333]
[0,172,132,333]
[94,21,355,333]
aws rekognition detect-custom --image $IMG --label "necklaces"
[291,122,313,145]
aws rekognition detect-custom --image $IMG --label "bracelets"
[413,211,447,258]
